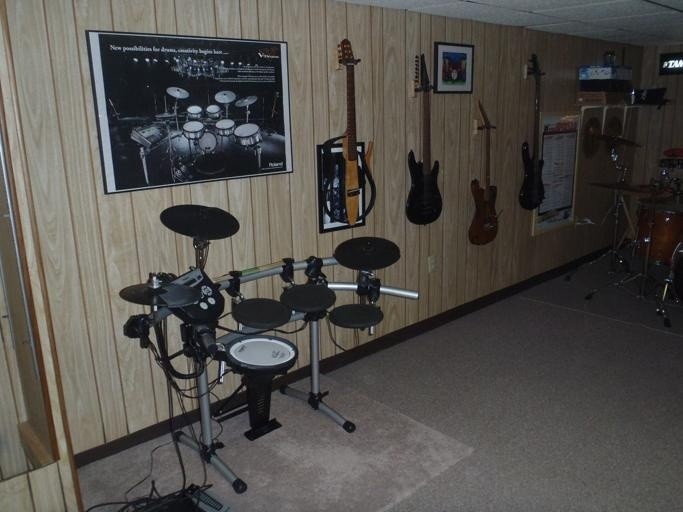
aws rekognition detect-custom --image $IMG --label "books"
[578,65,633,92]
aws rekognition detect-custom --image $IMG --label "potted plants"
[433,41,474,94]
[84,28,293,194]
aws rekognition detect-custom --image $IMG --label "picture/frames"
[585,183,673,301]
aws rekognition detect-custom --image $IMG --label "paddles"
[76,372,477,511]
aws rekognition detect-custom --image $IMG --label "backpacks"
[638,209,683,262]
[233,123,262,146]
[182,121,205,139]
[330,304,384,329]
[233,298,292,329]
[187,106,202,120]
[207,105,221,120]
[198,131,216,152]
[215,119,236,136]
[224,334,297,375]
[280,285,336,313]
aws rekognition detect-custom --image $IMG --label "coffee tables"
[334,237,400,270]
[235,95,257,107]
[586,179,650,192]
[159,204,239,239]
[120,283,204,308]
[166,87,190,99]
[215,90,237,103]
[599,134,643,147]
